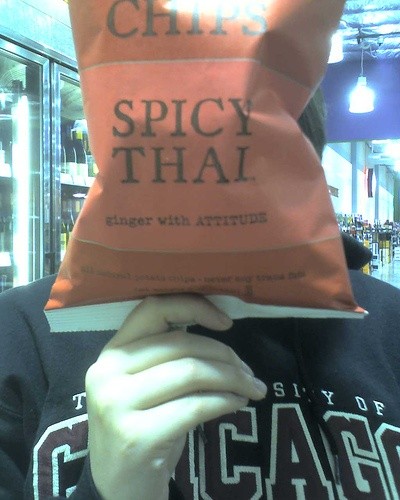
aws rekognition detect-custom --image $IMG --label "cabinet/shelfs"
[0,25,98,294]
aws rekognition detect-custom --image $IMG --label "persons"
[1,88,400,500]
[333,213,400,247]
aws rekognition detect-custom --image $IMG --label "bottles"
[61,123,97,179]
[61,196,82,266]
[338,212,399,275]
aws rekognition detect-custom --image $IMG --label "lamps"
[347,38,377,112]
